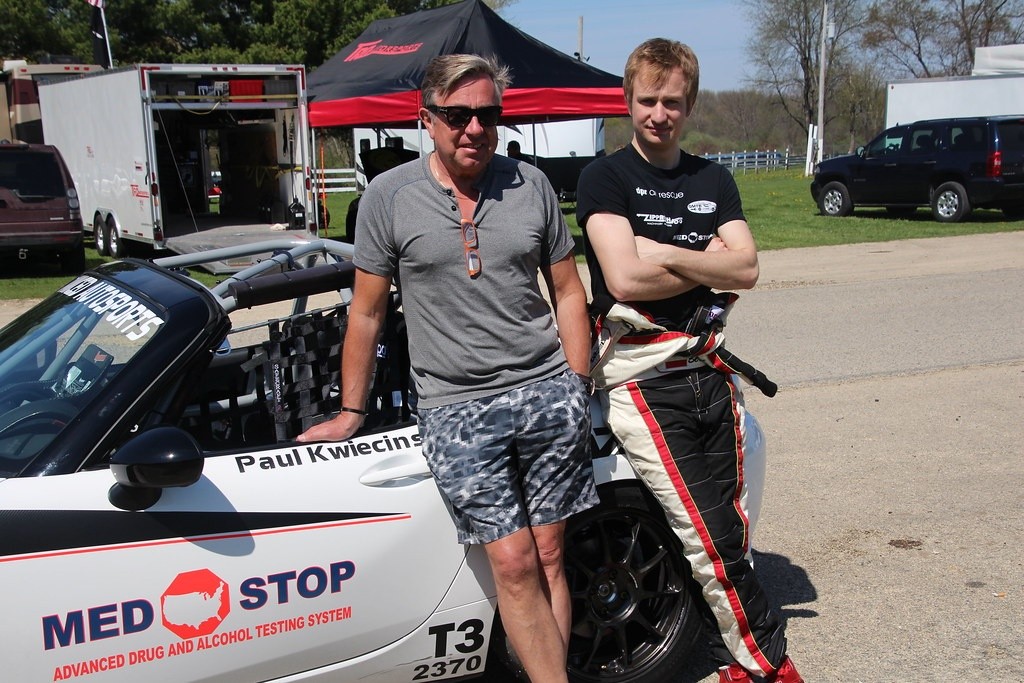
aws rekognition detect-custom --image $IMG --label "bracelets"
[340,407,368,416]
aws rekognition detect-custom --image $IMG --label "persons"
[507,140,539,167]
[575,37,806,683]
[293,54,601,683]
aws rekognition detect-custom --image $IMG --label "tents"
[304,0,630,237]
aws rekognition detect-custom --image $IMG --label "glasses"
[461,218,480,276]
[426,105,502,129]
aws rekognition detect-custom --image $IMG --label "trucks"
[1,58,318,275]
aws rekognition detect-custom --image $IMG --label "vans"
[0,139,86,269]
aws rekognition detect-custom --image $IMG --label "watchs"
[576,373,596,397]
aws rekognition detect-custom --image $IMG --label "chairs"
[238,292,405,445]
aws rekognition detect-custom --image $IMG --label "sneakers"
[765,655,804,683]
[718,662,756,683]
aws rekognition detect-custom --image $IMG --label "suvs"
[810,113,1024,224]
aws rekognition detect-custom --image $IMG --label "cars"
[0,233,768,683]
